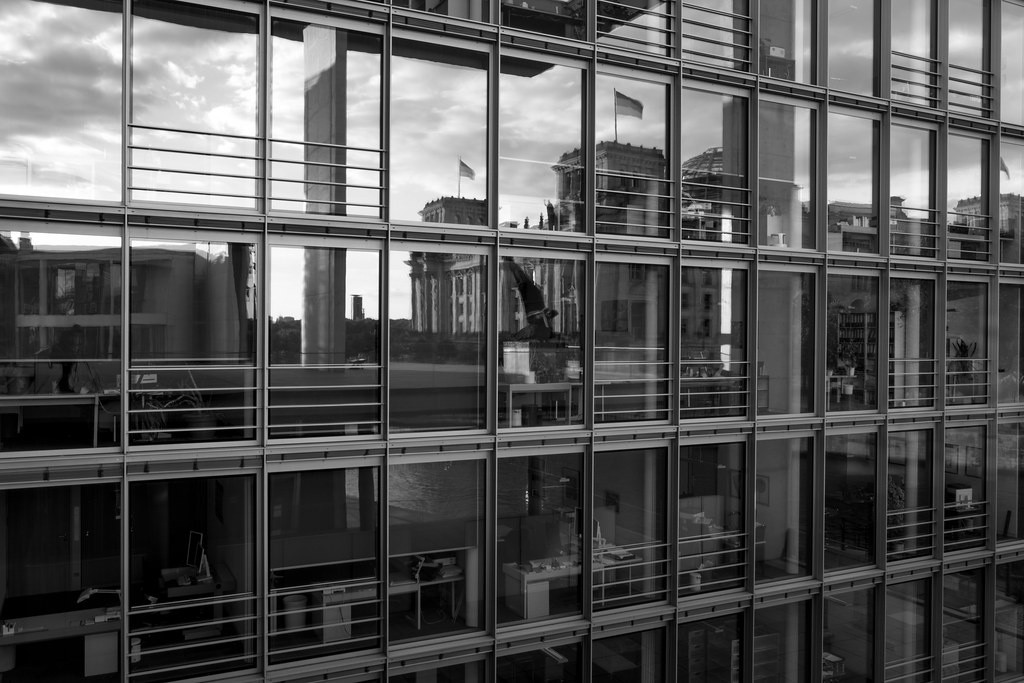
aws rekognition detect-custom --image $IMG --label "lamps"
[77,588,123,623]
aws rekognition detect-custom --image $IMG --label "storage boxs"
[947,487,973,513]
[116,374,157,390]
[768,47,785,58]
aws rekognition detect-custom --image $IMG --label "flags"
[615,91,644,119]
[460,161,475,180]
[1000,156,1010,178]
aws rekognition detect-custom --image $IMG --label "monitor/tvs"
[187,530,203,568]
[574,508,582,537]
[593,518,599,540]
[193,542,213,581]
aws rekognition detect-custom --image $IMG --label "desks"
[0,605,122,677]
[0,391,120,448]
[310,571,466,645]
[826,376,858,411]
[499,383,572,427]
[680,376,739,407]
[763,55,794,79]
[839,225,877,253]
[502,548,644,620]
[163,568,223,639]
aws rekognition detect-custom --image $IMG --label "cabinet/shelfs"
[837,311,905,406]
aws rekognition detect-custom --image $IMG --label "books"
[839,313,894,357]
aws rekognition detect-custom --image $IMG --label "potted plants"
[162,364,232,441]
[951,338,978,381]
[827,335,858,376]
[887,475,905,553]
[529,347,563,382]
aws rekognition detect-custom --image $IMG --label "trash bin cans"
[995,651,1007,673]
[689,573,702,591]
[131,637,141,663]
[513,409,522,426]
[284,595,308,629]
